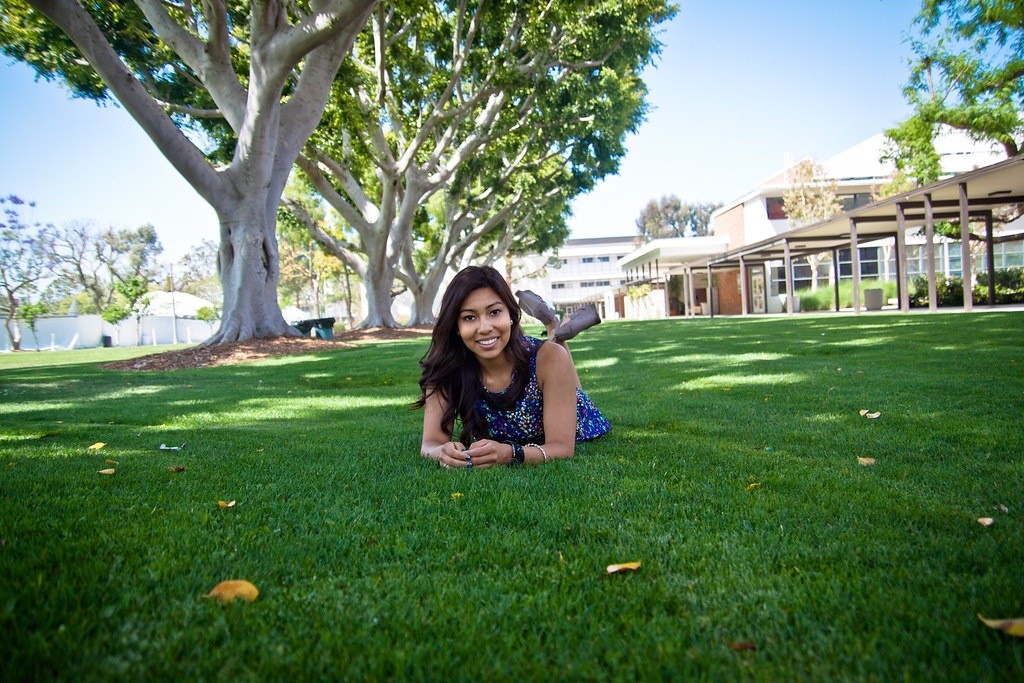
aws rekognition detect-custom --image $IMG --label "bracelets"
[504,440,525,467]
[525,443,546,463]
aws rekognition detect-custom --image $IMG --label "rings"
[444,463,448,468]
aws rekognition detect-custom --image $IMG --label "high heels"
[516,289,554,325]
[555,303,602,342]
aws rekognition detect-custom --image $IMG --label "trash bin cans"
[555,310,564,321]
[291,318,335,340]
[102,336,111,347]
[864,289,884,311]
[793,296,800,313]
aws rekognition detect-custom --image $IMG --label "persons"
[408,265,613,469]
